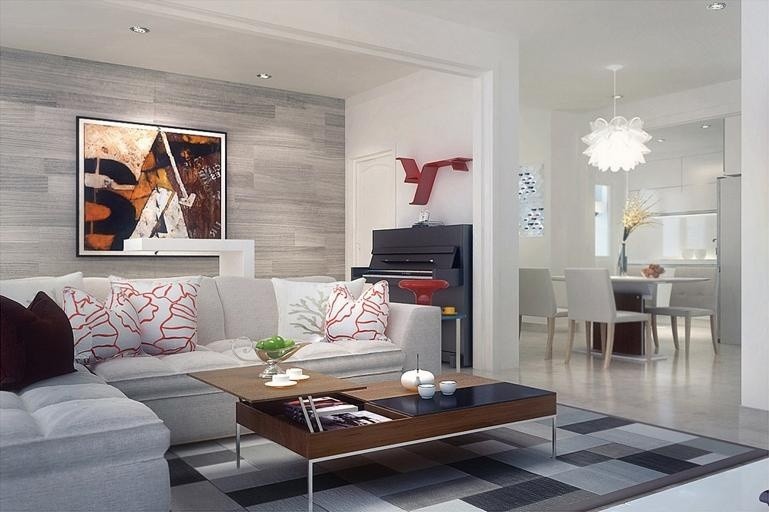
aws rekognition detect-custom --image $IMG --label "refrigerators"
[716,172,741,345]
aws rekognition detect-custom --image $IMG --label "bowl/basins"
[641,270,663,278]
[694,248,707,260]
[681,247,695,259]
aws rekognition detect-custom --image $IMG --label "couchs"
[0,274,441,512]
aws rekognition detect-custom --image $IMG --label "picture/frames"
[76,116,228,257]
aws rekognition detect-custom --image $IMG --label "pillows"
[213,276,339,342]
[105,275,202,355]
[272,277,367,344]
[60,286,143,364]
[0,270,82,311]
[1,291,77,394]
[108,277,225,348]
[322,280,392,343]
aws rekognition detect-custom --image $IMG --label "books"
[285,397,394,431]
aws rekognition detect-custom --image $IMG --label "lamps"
[579,65,654,173]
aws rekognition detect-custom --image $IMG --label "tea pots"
[400,353,434,392]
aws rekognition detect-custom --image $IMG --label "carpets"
[164,403,768,511]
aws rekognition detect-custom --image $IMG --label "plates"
[286,374,309,380]
[265,381,298,387]
[441,312,457,315]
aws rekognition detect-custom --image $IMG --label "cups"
[441,306,455,313]
[417,383,436,400]
[440,379,457,396]
[286,367,302,377]
[272,374,288,383]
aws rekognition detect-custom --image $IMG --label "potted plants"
[614,188,665,277]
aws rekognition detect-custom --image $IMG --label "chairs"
[519,268,575,363]
[644,266,721,354]
[398,280,467,373]
[618,264,658,354]
[562,267,653,370]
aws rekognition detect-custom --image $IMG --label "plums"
[650,264,664,277]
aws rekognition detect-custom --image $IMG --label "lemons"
[255,335,294,358]
